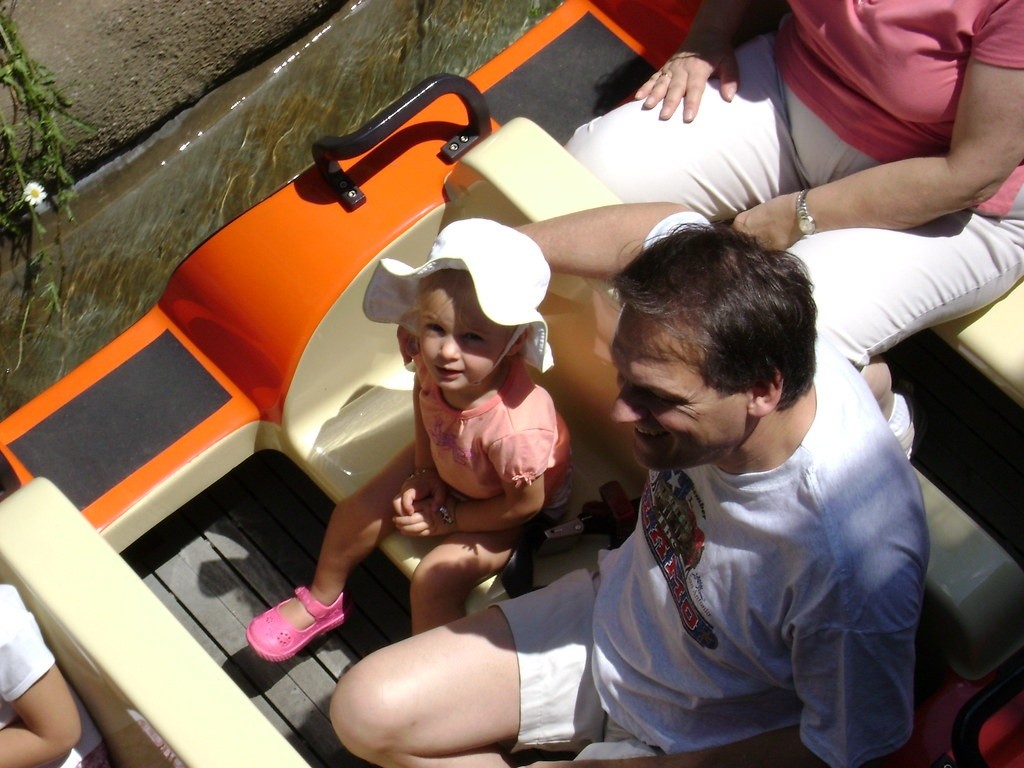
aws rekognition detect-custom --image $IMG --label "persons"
[560,1,1024,465]
[245,217,558,683]
[326,219,930,768]
[0,581,113,768]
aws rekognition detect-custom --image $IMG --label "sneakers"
[895,379,928,464]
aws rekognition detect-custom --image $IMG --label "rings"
[659,73,673,80]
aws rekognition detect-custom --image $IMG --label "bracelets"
[405,467,435,481]
[452,497,460,532]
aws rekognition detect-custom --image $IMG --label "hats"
[361,218,555,377]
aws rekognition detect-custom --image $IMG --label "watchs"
[795,186,817,238]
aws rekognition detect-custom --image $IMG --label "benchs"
[0,478,322,767]
[279,118,1024,768]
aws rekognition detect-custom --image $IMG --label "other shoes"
[245,585,347,661]
